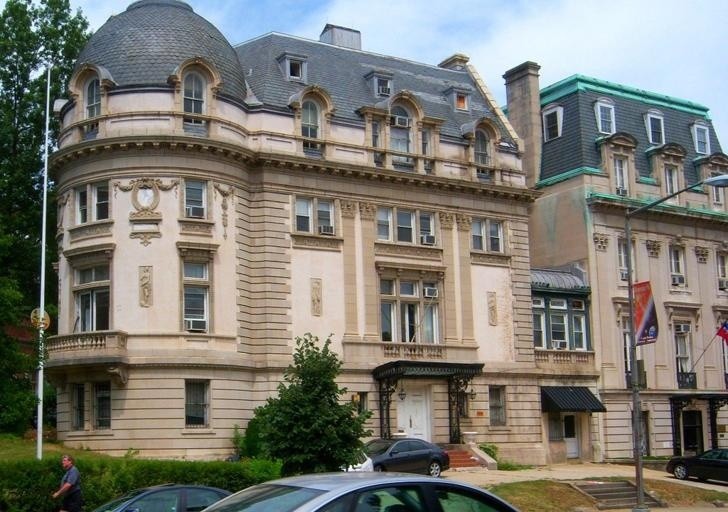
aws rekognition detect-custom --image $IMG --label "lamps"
[398,377,406,400]
[469,378,476,400]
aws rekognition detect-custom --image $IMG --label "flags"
[716,318,728,344]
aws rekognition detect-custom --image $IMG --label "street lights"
[625,174,728,511]
[35,53,57,461]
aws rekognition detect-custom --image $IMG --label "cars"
[665,447,728,480]
[338,437,450,478]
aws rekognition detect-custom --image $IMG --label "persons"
[50,453,82,512]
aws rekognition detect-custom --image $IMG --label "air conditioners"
[186,319,208,333]
[617,188,628,197]
[319,224,439,298]
[620,271,728,335]
[378,86,409,128]
[552,340,567,349]
